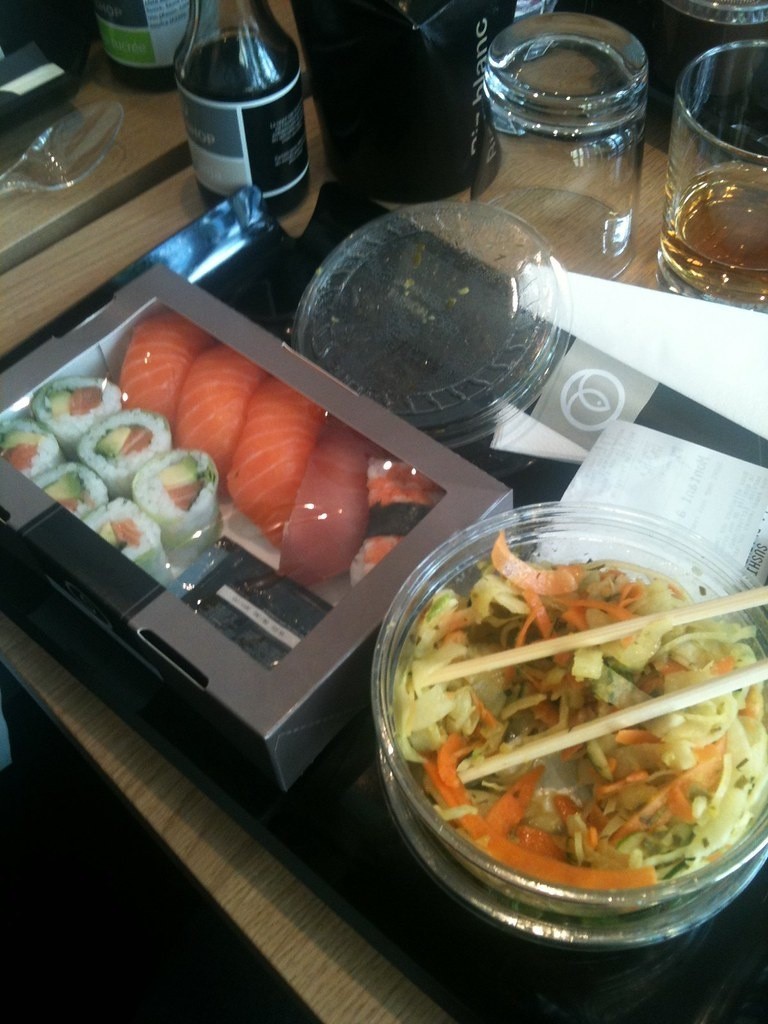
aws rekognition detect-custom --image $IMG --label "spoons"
[0,99,124,196]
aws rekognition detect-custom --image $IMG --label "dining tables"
[0,3,768,1024]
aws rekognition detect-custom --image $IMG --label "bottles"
[94,0,220,93]
[291,0,502,211]
[173,0,312,217]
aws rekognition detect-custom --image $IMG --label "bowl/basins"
[371,501,768,947]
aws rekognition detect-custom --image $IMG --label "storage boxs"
[0,254,513,801]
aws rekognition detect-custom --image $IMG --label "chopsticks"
[407,585,768,795]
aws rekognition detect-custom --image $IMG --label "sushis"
[0,303,439,593]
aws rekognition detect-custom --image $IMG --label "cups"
[471,12,648,281]
[655,40,768,314]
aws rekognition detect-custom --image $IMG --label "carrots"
[418,531,734,891]
